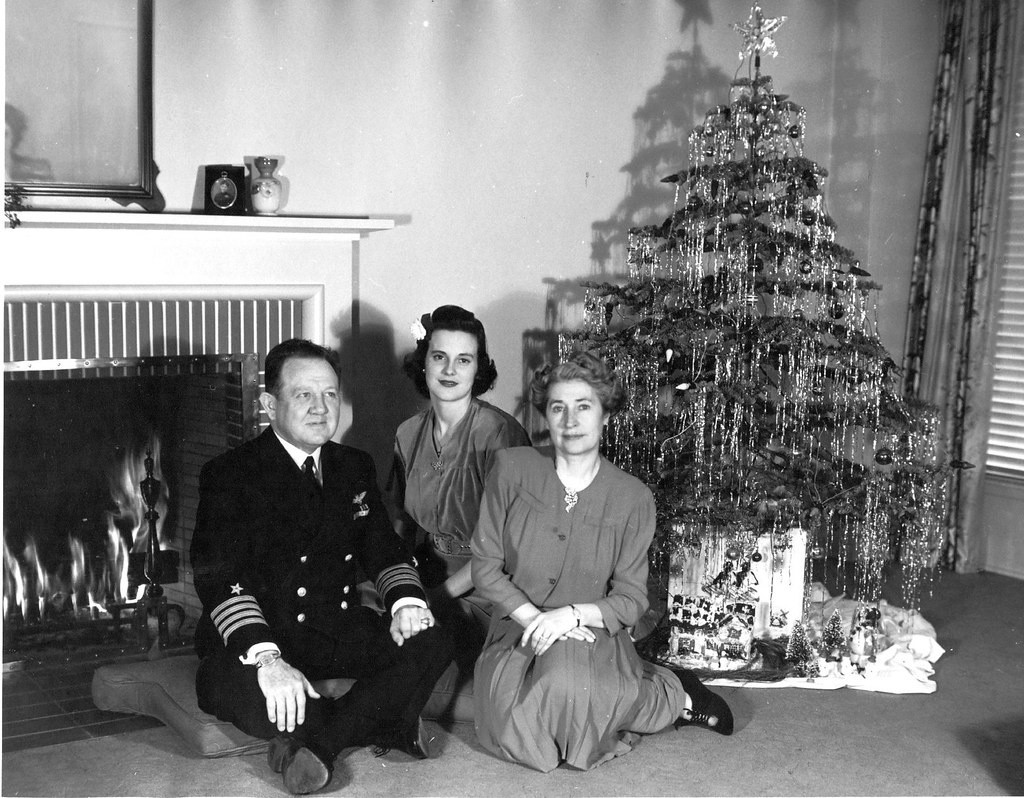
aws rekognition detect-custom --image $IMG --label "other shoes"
[268,734,336,794]
[367,714,429,758]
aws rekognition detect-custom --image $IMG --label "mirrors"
[5,0,155,200]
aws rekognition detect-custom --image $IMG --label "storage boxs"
[667,521,807,642]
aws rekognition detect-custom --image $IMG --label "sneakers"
[672,670,733,736]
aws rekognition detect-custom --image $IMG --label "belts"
[424,532,472,556]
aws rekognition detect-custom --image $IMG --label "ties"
[304,456,318,482]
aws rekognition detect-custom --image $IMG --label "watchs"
[569,603,582,628]
[255,652,280,669]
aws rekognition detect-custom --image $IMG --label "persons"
[387,305,534,656]
[469,352,734,773]
[189,339,458,796]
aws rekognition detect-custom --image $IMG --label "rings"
[421,617,430,626]
[541,636,548,640]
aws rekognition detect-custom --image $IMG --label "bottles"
[252,157,283,216]
